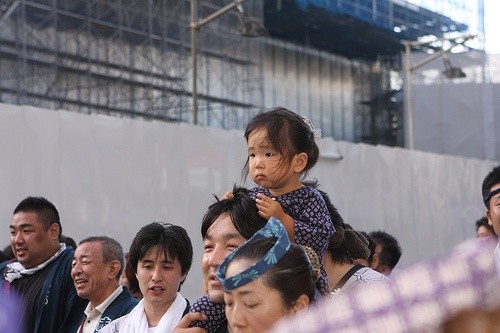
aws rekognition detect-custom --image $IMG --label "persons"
[188,105,332,333]
[0,168,500,333]
